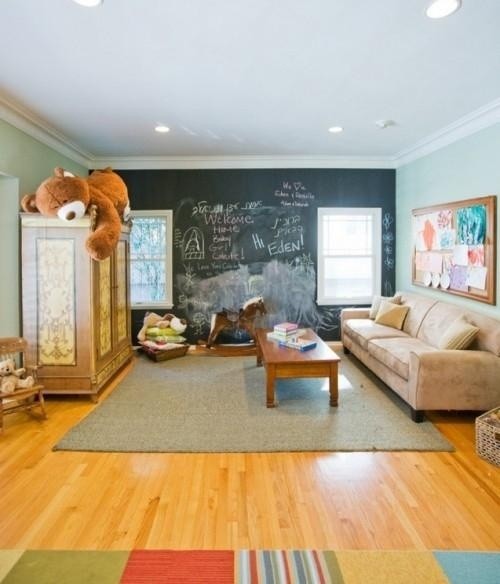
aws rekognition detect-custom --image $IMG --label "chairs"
[0,337,49,427]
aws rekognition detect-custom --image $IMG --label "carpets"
[52,352,457,453]
[0,550,500,584]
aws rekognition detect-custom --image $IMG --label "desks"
[254,327,341,408]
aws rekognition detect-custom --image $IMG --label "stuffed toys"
[135,308,189,343]
[0,356,35,393]
[21,164,131,264]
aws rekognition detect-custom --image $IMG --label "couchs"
[340,290,500,423]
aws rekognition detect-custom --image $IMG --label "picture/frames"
[411,194,497,306]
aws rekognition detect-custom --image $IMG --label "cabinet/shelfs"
[18,212,134,404]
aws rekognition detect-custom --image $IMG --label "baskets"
[474,406,500,469]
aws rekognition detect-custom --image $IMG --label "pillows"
[375,300,410,330]
[437,313,480,350]
[370,294,402,320]
[145,336,188,343]
[146,326,183,335]
[138,340,187,350]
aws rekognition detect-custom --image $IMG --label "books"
[265,321,318,351]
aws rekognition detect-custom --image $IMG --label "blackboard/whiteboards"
[88,169,396,344]
